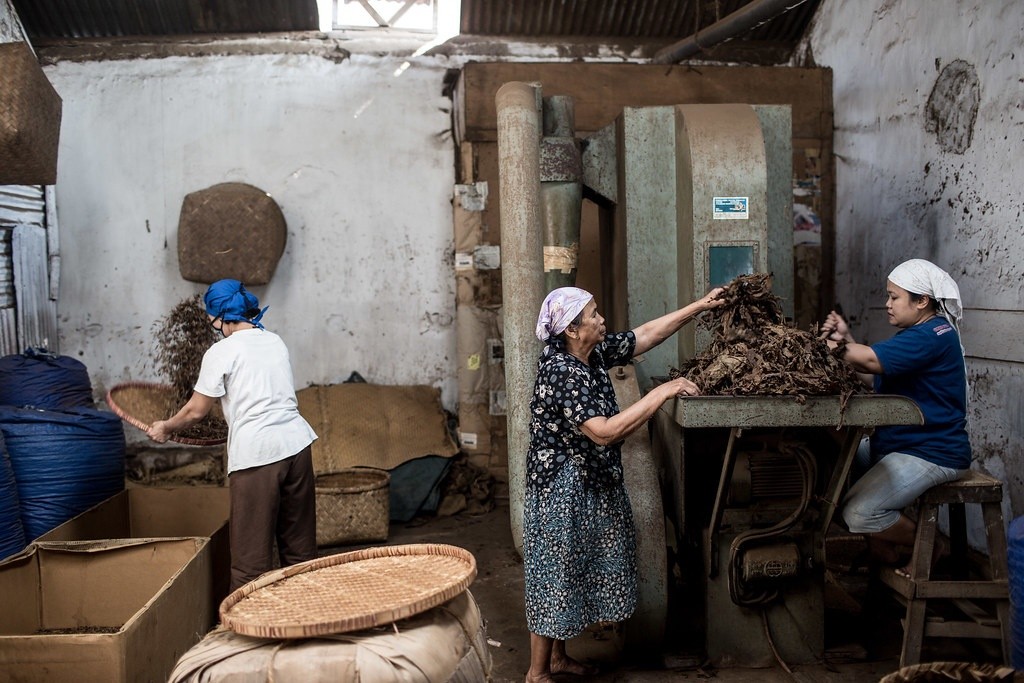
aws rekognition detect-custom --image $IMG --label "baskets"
[315,467,392,544]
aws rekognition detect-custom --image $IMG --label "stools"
[836,471,1014,671]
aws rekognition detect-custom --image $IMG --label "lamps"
[317,0,462,50]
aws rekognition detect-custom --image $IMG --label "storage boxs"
[0,458,233,683]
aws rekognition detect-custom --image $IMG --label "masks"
[209,308,227,342]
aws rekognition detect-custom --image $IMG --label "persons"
[524,284,730,683]
[146,279,320,593]
[819,259,972,579]
